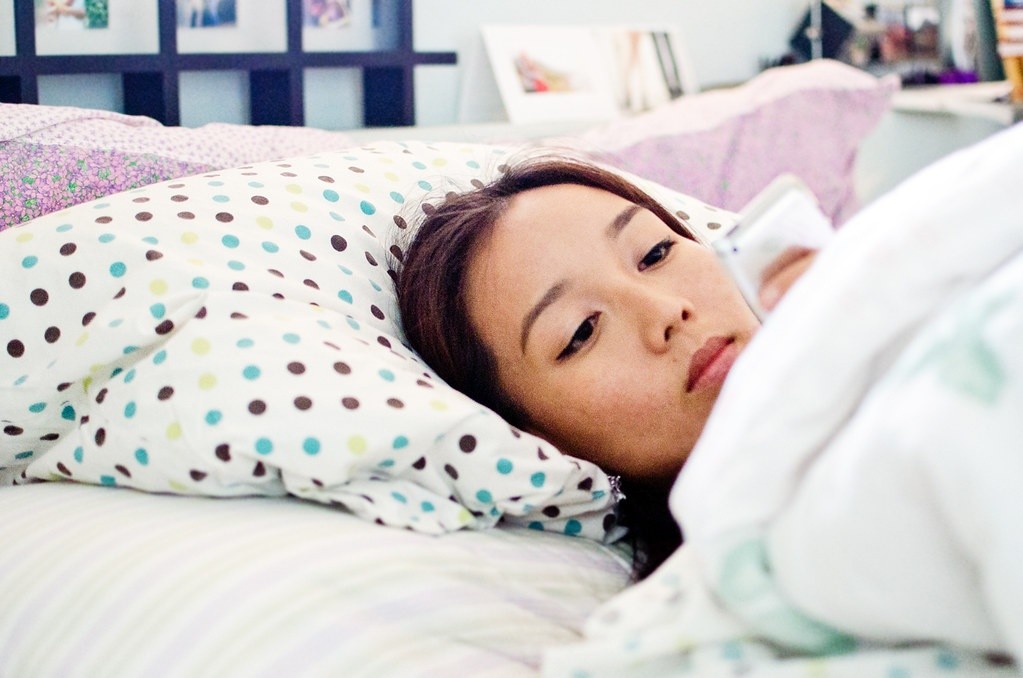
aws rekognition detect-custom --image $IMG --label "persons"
[392,145,824,589]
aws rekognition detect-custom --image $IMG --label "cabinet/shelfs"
[0,1,460,126]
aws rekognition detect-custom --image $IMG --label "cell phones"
[707,170,839,323]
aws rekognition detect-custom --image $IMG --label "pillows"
[0,63,901,231]
[1,146,743,534]
